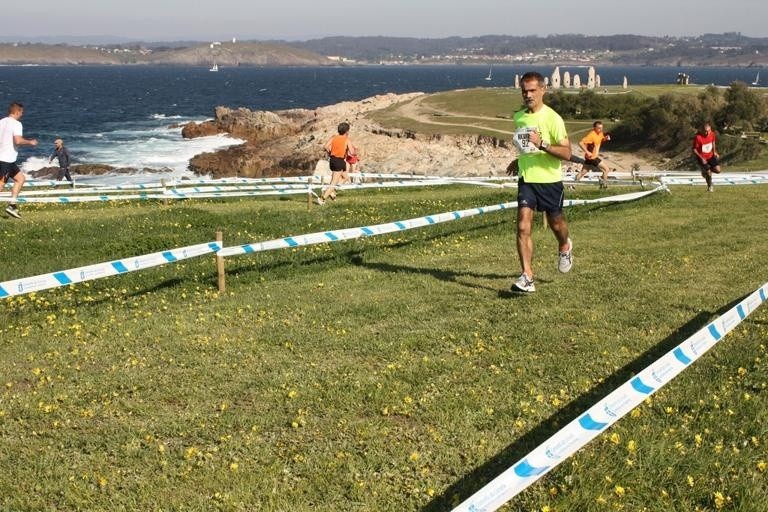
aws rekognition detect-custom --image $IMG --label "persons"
[568,122,610,192]
[504,72,574,293]
[48,138,75,189]
[0,103,39,219]
[692,122,721,191]
[316,122,360,207]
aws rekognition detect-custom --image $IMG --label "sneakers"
[511,272,535,292]
[6,204,22,219]
[316,196,325,206]
[558,238,573,274]
[329,192,336,202]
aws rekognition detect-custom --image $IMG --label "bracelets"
[539,140,549,151]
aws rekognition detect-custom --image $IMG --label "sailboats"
[752,72,761,86]
[209,63,218,72]
[484,67,492,80]
